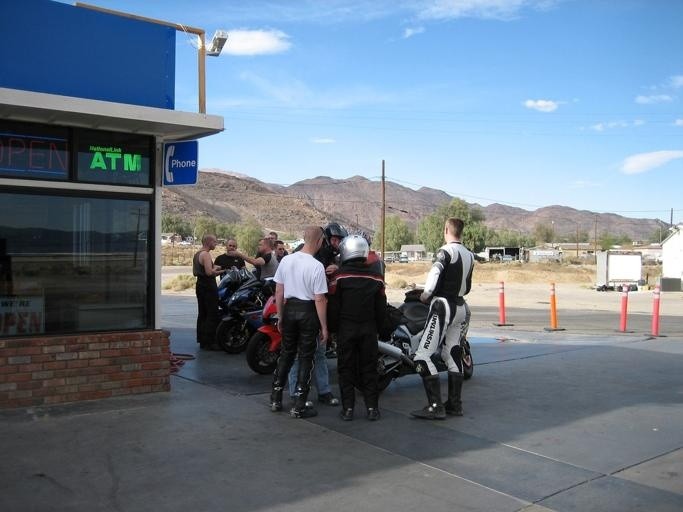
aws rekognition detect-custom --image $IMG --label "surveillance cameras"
[209,32,227,56]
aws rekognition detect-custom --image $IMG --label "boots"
[287,385,318,418]
[409,373,446,420]
[270,385,283,412]
[443,371,465,417]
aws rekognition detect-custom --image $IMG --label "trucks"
[596,249,643,292]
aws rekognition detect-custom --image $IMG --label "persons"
[194,223,386,420]
[411,219,475,420]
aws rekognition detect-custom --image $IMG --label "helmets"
[321,222,348,256]
[338,234,369,266]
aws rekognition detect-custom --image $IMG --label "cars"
[384,257,395,263]
[397,255,408,263]
[179,241,192,246]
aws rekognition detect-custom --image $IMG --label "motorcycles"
[209,264,339,379]
[354,289,473,393]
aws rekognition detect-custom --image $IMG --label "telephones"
[165,145,176,183]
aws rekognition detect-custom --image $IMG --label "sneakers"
[365,407,380,420]
[199,342,223,352]
[338,407,354,422]
[318,393,340,406]
